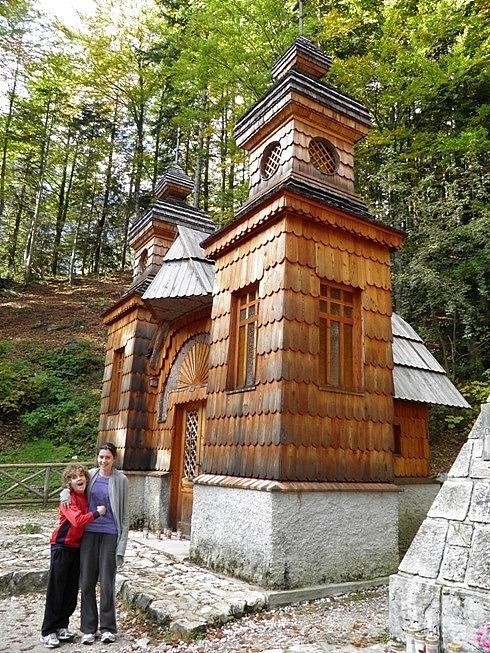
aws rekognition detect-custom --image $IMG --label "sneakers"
[100,631,116,642]
[56,627,74,640]
[39,632,60,648]
[81,633,96,644]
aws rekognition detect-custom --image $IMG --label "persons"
[39,463,106,649]
[59,442,129,647]
